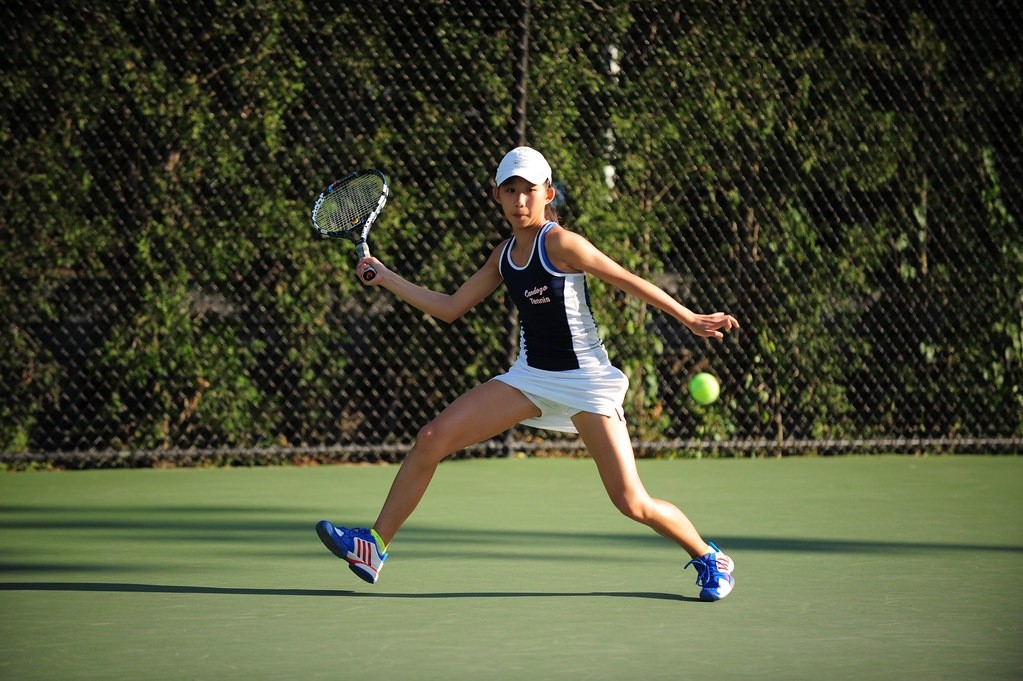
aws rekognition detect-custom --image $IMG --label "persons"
[315,145,740,603]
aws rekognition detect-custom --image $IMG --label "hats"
[495,146,552,187]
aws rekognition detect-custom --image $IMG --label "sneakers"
[316,520,388,584]
[684,541,736,602]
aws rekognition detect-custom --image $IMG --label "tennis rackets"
[309,167,389,281]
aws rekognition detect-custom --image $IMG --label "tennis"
[689,372,719,404]
